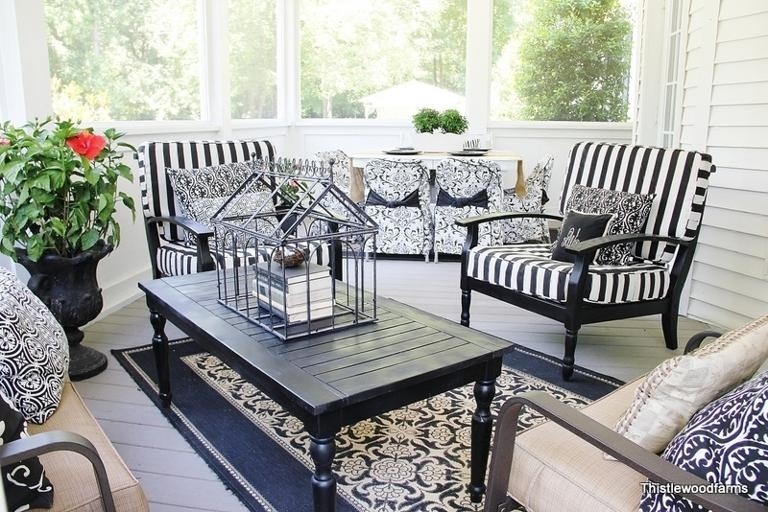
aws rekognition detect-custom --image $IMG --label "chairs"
[0,373,152,512]
[454,142,716,383]
[483,332,767,510]
[133,140,342,282]
[318,150,555,265]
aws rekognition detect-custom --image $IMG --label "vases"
[13,240,114,381]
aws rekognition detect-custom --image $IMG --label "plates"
[461,147,493,151]
[382,146,423,155]
[449,150,490,156]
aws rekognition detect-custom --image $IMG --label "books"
[252,259,334,324]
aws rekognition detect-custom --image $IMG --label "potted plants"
[411,109,470,152]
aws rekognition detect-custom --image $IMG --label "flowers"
[1,115,138,264]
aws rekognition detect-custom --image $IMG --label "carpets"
[110,337,627,512]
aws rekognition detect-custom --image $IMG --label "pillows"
[602,313,767,463]
[0,265,70,424]
[164,158,284,248]
[551,183,657,267]
